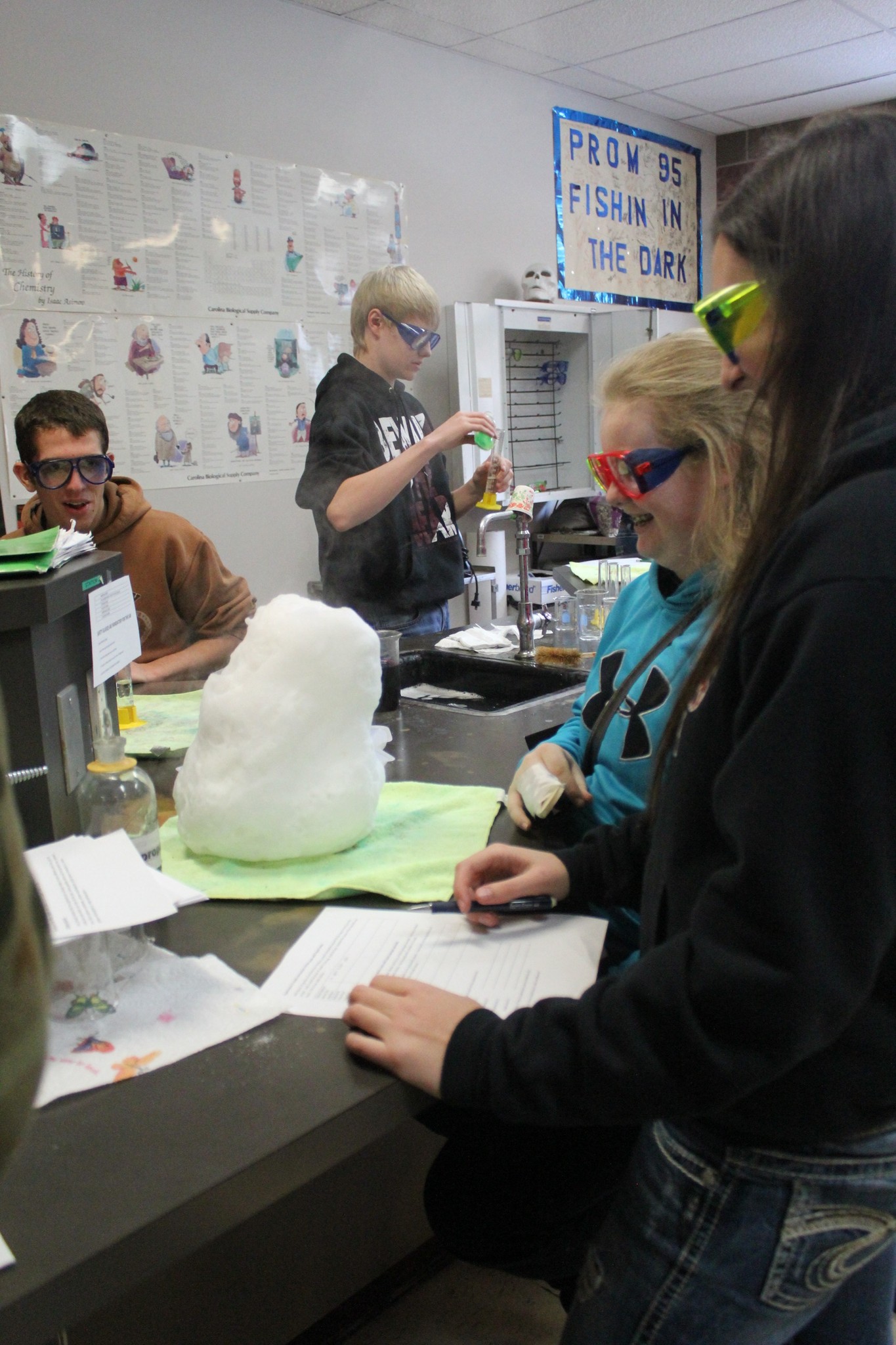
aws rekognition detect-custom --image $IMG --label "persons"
[295,266,513,637]
[504,327,789,830]
[343,107,896,1345]
[0,390,256,685]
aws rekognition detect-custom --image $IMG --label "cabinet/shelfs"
[451,298,657,505]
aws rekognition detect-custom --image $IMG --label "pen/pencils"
[409,895,558,914]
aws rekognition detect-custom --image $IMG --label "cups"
[505,485,534,523]
[375,630,403,713]
[603,597,618,624]
[553,596,577,631]
[574,589,609,641]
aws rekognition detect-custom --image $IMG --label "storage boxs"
[506,568,570,605]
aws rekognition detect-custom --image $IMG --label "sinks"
[396,646,590,715]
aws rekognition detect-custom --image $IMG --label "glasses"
[383,313,440,350]
[692,272,777,367]
[25,454,115,489]
[587,437,710,501]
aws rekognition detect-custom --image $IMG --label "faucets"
[476,510,535,662]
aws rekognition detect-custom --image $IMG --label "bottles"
[115,664,137,725]
[47,923,149,1023]
[75,737,161,872]
[474,411,496,451]
